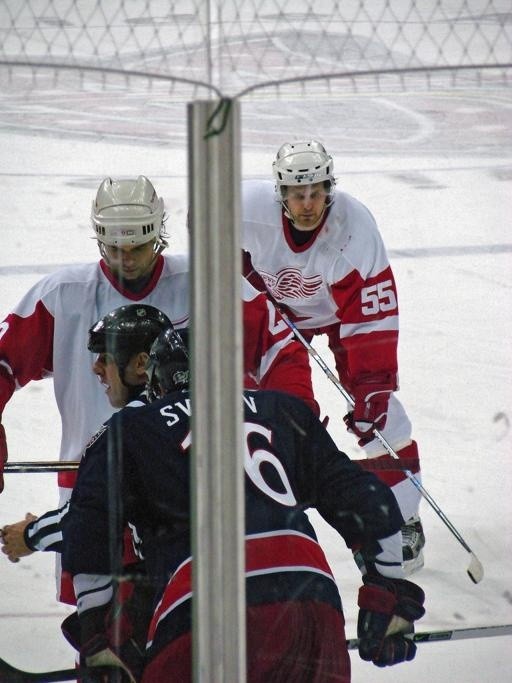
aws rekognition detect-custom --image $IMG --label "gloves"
[59,579,145,683]
[355,574,426,667]
[341,380,394,448]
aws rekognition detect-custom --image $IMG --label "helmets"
[271,135,334,187]
[85,302,173,369]
[91,175,165,250]
[146,329,191,394]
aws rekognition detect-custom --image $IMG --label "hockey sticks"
[273,303,482,584]
[1,624,512,682]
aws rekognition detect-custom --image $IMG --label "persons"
[56,316,433,682]
[0,301,177,676]
[0,171,323,642]
[232,135,427,574]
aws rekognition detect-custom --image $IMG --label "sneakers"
[399,511,426,561]
